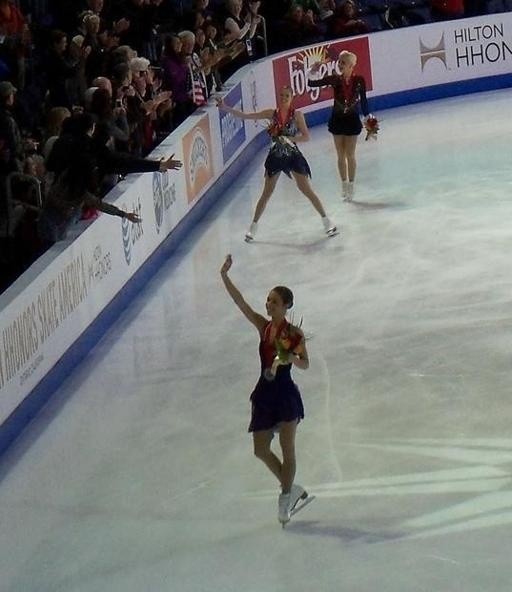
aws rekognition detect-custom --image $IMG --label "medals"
[263,368,274,380]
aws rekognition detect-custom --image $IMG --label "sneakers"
[278,494,291,522]
[323,218,336,234]
[245,221,257,240]
[340,180,355,198]
[290,484,308,511]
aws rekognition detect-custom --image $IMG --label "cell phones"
[115,99,122,108]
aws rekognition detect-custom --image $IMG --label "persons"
[219,253,318,529]
